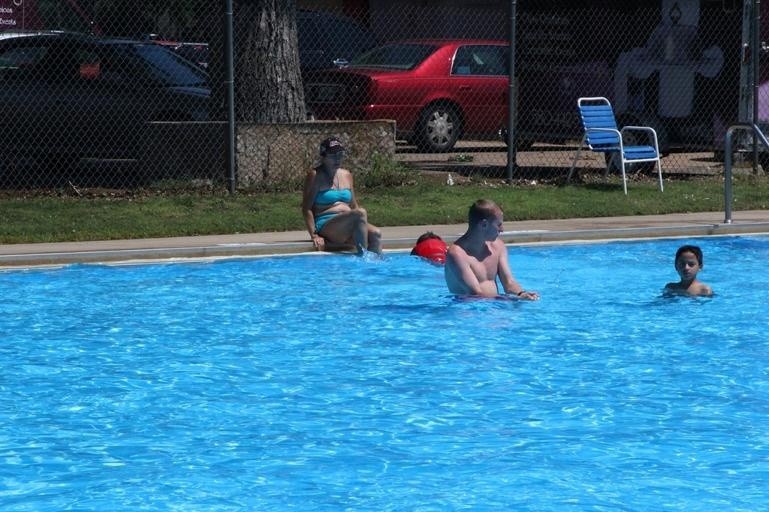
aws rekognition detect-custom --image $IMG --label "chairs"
[568,96,666,194]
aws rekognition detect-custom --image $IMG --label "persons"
[445,198,539,302]
[302,137,382,255]
[416,230,442,245]
[665,244,715,296]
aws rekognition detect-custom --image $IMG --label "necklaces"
[322,167,340,190]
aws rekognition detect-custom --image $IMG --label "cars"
[208,7,389,85]
[0,30,224,184]
[303,38,535,152]
[17,34,208,78]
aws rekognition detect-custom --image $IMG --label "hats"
[320,138,343,154]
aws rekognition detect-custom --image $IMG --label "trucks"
[506,1,769,176]
